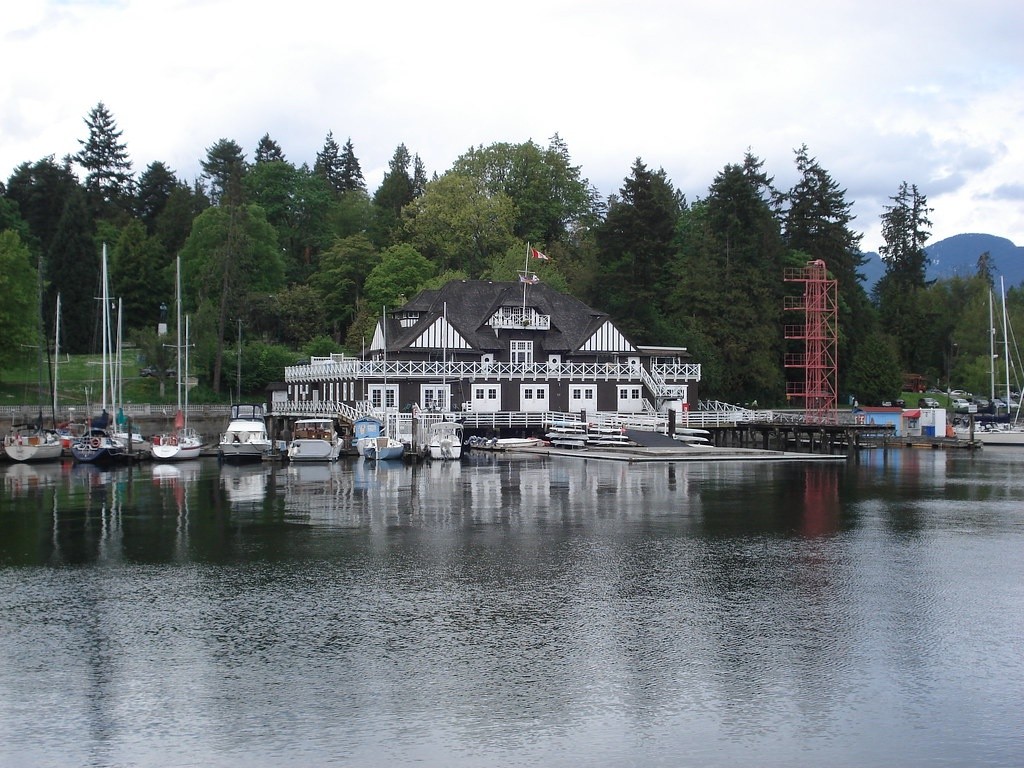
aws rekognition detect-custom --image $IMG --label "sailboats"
[69,242,154,464]
[429,301,464,461]
[363,305,405,461]
[2,255,63,462]
[952,276,1024,447]
[150,256,203,462]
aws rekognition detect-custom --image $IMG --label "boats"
[468,437,542,450]
[286,418,343,461]
[218,403,270,458]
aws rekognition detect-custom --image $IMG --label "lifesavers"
[91,438,100,447]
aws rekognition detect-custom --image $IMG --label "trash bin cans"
[922,425,935,436]
[682,403,691,423]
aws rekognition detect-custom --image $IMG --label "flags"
[526,246,548,260]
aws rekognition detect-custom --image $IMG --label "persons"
[852,397,859,412]
[752,400,758,412]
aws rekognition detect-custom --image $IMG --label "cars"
[951,399,969,408]
[919,398,939,409]
[891,399,905,407]
[1001,391,1021,409]
[974,399,989,410]
[950,390,972,398]
[987,399,1007,408]
[925,389,948,396]
